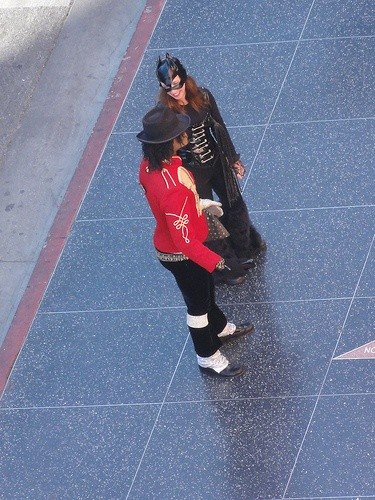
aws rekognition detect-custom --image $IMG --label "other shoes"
[224,274,244,284]
[259,239,266,249]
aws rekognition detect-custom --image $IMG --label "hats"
[136,107,190,144]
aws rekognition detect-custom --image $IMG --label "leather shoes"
[199,362,246,379]
[219,322,254,343]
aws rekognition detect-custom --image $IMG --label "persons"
[137,106,255,378]
[155,52,266,286]
